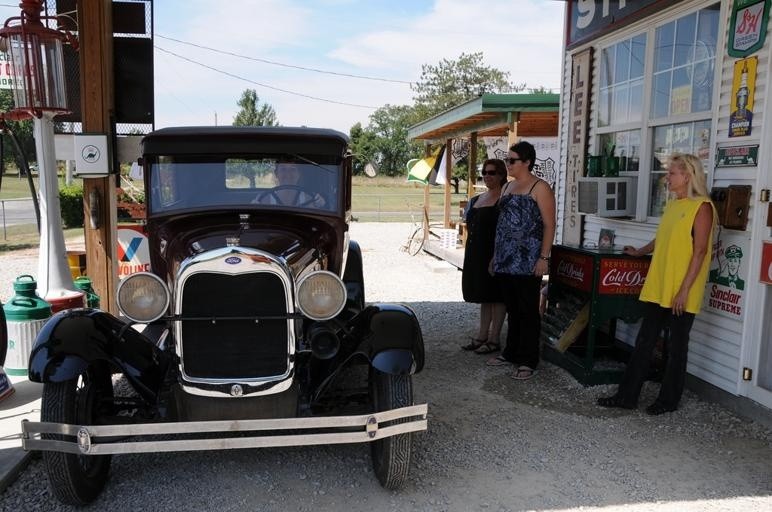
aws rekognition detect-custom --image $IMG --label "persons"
[486,140,555,379]
[708,243,744,290]
[596,153,720,414]
[249,159,326,208]
[459,159,508,355]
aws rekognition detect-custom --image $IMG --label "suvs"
[11,123,430,507]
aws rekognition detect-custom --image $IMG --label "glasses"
[482,170,496,175]
[504,159,521,164]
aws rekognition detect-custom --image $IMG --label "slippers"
[511,368,540,379]
[486,356,513,366]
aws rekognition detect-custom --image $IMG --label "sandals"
[476,342,501,354]
[463,338,487,351]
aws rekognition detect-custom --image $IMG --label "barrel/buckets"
[44,289,87,315]
[2,275,51,376]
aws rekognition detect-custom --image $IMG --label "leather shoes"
[646,403,677,415]
[598,394,637,409]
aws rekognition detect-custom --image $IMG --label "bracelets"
[539,255,549,261]
